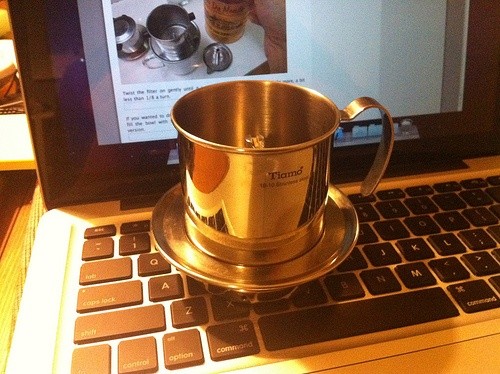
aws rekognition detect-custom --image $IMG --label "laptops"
[4,0,500,374]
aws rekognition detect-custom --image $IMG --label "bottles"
[204,0,251,44]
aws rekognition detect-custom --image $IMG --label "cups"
[146,4,195,55]
[169,79,395,264]
[113,14,142,55]
[142,21,201,75]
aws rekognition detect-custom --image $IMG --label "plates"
[149,182,360,292]
[117,24,149,61]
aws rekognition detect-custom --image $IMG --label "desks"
[0,159,500,370]
